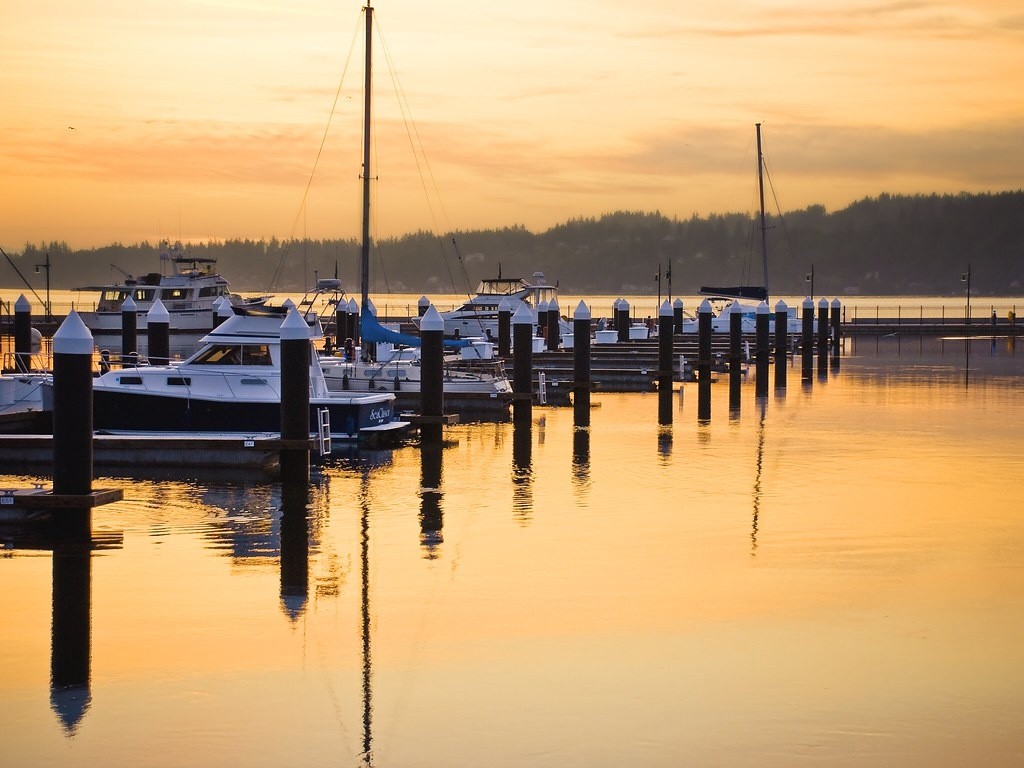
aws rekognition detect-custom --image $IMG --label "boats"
[64,0,838,410]
[92,280,412,447]
[1,353,55,435]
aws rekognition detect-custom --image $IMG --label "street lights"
[806,264,814,302]
[653,265,660,309]
[33,254,54,324]
[664,258,672,305]
[960,263,970,324]
